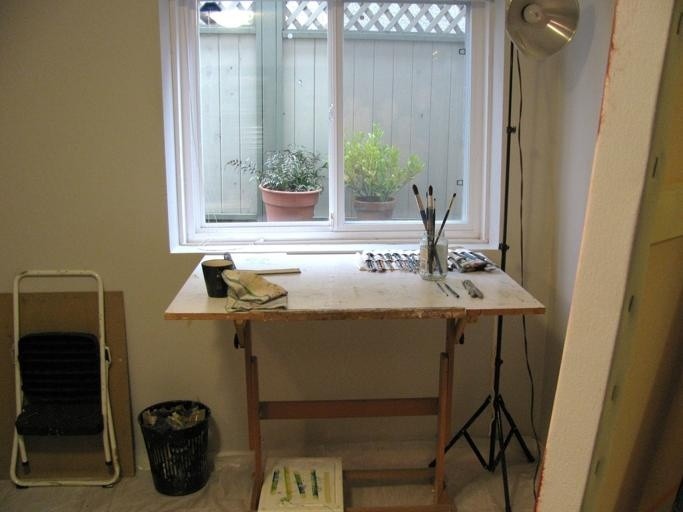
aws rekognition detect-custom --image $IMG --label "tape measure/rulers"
[244,267,302,276]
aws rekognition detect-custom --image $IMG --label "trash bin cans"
[138,400,210,496]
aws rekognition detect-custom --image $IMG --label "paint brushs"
[412,184,457,274]
[365,252,488,272]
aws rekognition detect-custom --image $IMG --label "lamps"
[424,0,584,510]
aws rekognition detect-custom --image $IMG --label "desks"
[165,251,545,512]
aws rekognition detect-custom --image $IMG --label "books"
[229,252,302,275]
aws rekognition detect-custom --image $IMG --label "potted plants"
[343,122,426,221]
[224,145,329,222]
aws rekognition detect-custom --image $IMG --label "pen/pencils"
[445,283,460,298]
[462,280,484,298]
[437,282,449,297]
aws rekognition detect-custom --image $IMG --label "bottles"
[417,231,448,281]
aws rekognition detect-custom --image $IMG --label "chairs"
[9,270,122,490]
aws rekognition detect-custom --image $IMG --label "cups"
[200,258,233,297]
